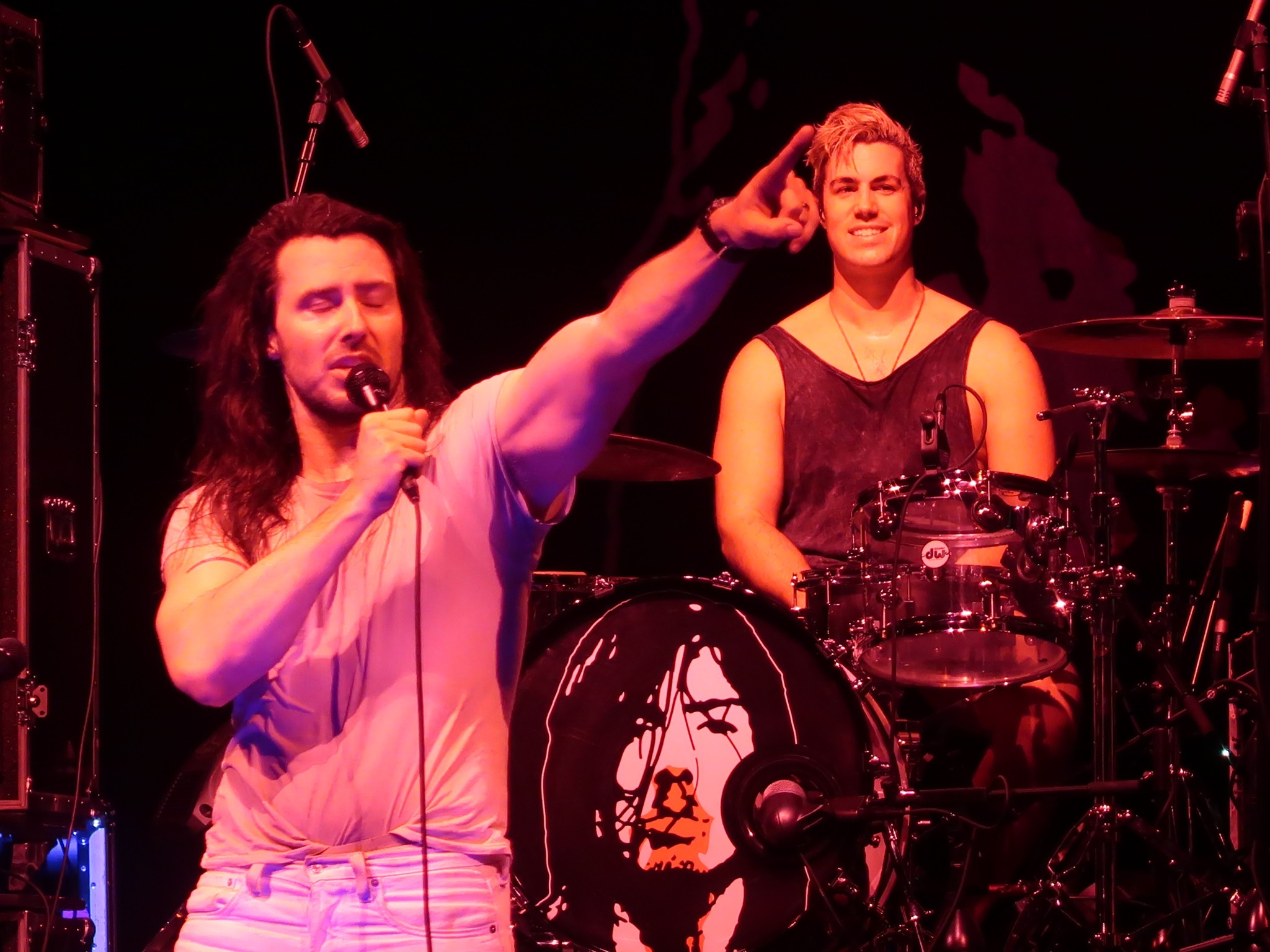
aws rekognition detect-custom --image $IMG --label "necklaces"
[829,285,925,383]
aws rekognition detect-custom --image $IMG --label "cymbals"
[1018,306,1264,359]
[576,433,723,483]
[1055,447,1256,488]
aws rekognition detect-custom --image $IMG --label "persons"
[712,101,1083,952]
[155,124,820,952]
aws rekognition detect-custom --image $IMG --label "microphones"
[936,393,950,470]
[758,779,834,846]
[284,8,369,149]
[343,362,421,503]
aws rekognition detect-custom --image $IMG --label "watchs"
[699,196,766,264]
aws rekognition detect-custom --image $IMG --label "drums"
[507,577,910,952]
[794,563,1018,651]
[849,468,1076,693]
[520,569,641,645]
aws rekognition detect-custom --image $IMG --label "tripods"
[1002,325,1270,952]
